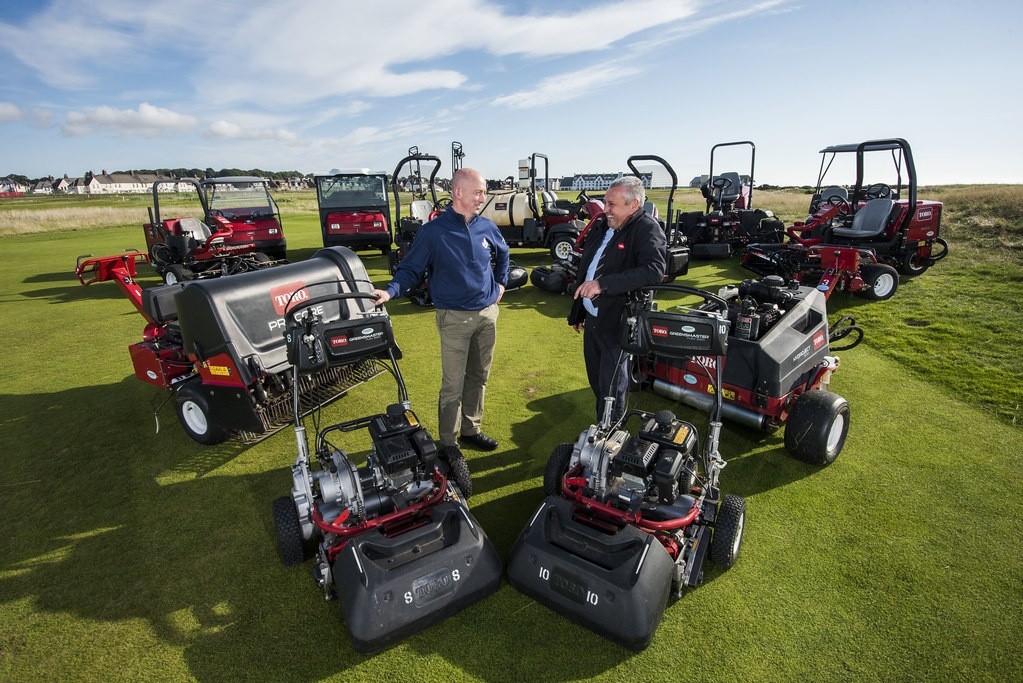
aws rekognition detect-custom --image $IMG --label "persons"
[567,176,667,431]
[369,168,510,460]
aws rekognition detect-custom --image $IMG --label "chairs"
[410,200,438,226]
[868,187,892,199]
[713,172,741,202]
[821,185,848,202]
[542,191,569,215]
[179,218,212,245]
[832,199,893,238]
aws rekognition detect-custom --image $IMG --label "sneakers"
[461,433,498,450]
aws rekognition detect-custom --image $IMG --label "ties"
[590,230,618,307]
